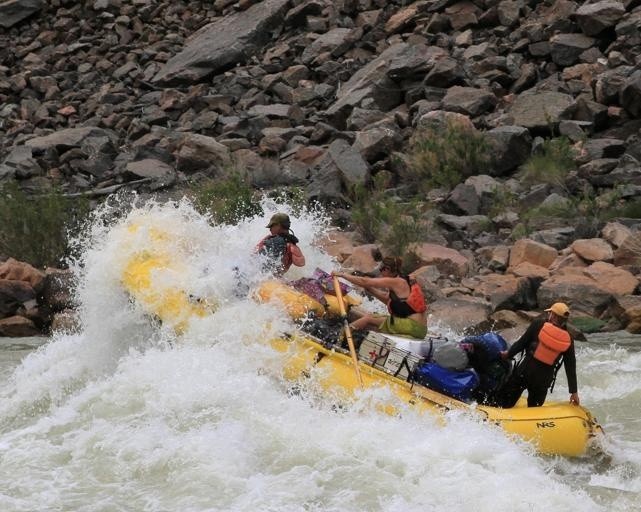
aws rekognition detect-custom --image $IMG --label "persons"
[329,251,429,347]
[249,212,306,277]
[495,302,581,409]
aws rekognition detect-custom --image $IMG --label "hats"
[544,303,569,318]
[265,213,291,229]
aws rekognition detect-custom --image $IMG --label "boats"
[121,224,610,471]
